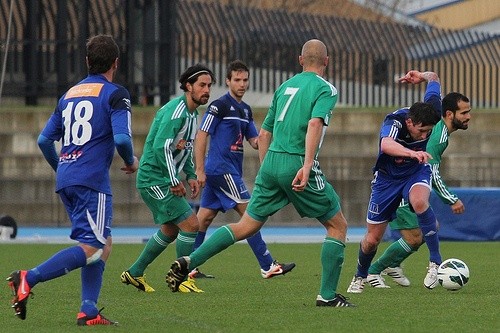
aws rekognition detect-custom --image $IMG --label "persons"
[188,60,296,279]
[164,39,357,308]
[346,70,443,292]
[367,92,471,287]
[120,63,201,292]
[6,34,139,326]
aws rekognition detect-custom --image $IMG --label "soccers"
[437,258,472,292]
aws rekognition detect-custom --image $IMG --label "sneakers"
[188,268,215,278]
[366,273,391,288]
[77,307,115,325]
[5,269,35,320]
[179,276,205,293]
[347,275,367,294]
[261,260,296,279]
[424,261,439,289]
[120,269,157,292]
[381,267,410,287]
[166,256,190,292]
[315,294,359,307]
